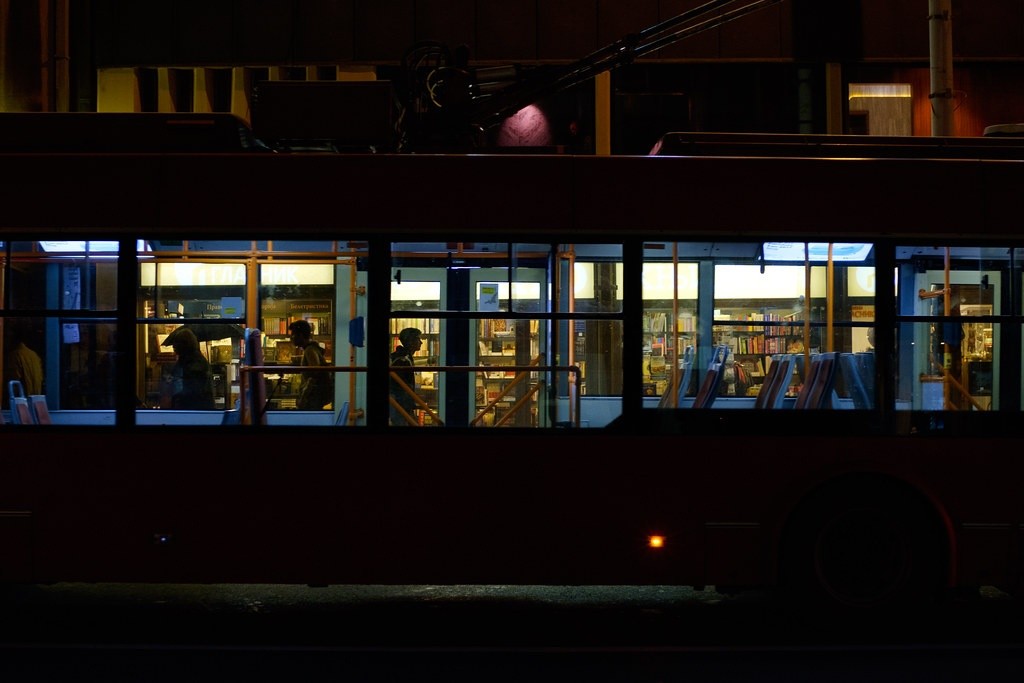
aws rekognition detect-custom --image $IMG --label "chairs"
[968,361,993,413]
[9,380,52,426]
[659,343,875,412]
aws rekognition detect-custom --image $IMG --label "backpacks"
[312,360,334,406]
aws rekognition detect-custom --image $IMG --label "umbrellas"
[161,324,245,362]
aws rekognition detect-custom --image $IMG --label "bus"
[0,75,1024,588]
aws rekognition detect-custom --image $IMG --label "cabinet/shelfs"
[142,296,332,410]
[642,306,821,401]
[390,304,540,427]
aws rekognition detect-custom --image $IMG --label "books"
[262,318,331,409]
[146,334,245,410]
[643,312,801,397]
[389,318,538,425]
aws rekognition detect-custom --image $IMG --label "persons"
[289,320,328,411]
[390,328,423,425]
[9,325,43,396]
[722,360,753,397]
[172,328,214,410]
